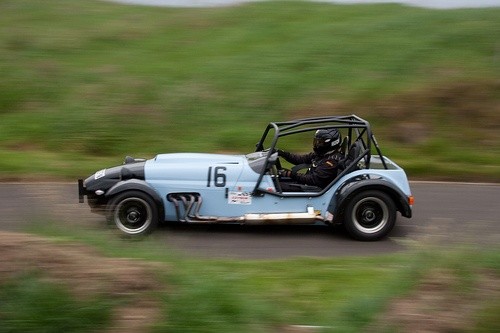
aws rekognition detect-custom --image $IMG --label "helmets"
[313,128,342,152]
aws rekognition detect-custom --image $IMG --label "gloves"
[271,148,289,159]
[278,168,290,177]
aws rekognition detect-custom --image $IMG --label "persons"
[268,128,344,192]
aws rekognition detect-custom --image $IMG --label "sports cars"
[78,114,415,243]
